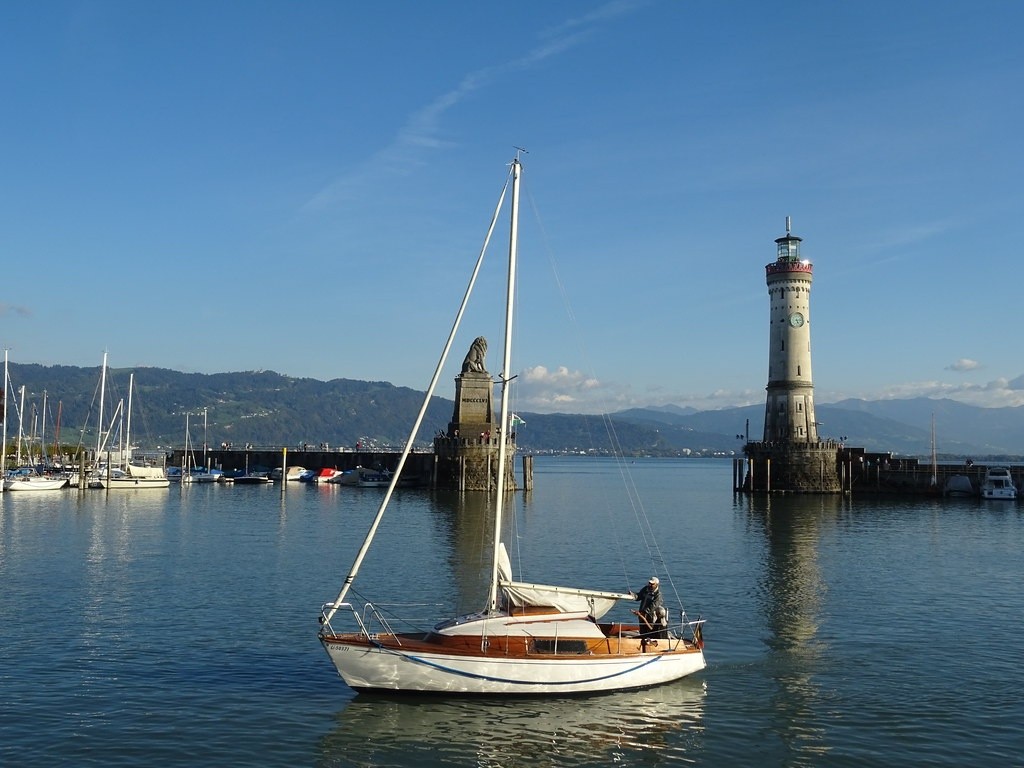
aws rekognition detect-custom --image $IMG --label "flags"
[512,414,527,428]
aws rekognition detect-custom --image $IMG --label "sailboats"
[0,347,222,491]
[317,150,708,699]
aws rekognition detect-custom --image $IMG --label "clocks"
[791,314,803,326]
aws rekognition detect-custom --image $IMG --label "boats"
[944,474,977,499]
[979,467,1017,500]
[233,464,397,487]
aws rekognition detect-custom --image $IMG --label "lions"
[461,335,490,373]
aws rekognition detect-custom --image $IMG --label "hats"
[648,577,659,584]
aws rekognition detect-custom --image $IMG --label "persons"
[303,442,307,452]
[630,575,668,639]
[221,440,234,451]
[478,428,502,444]
[355,440,361,453]
